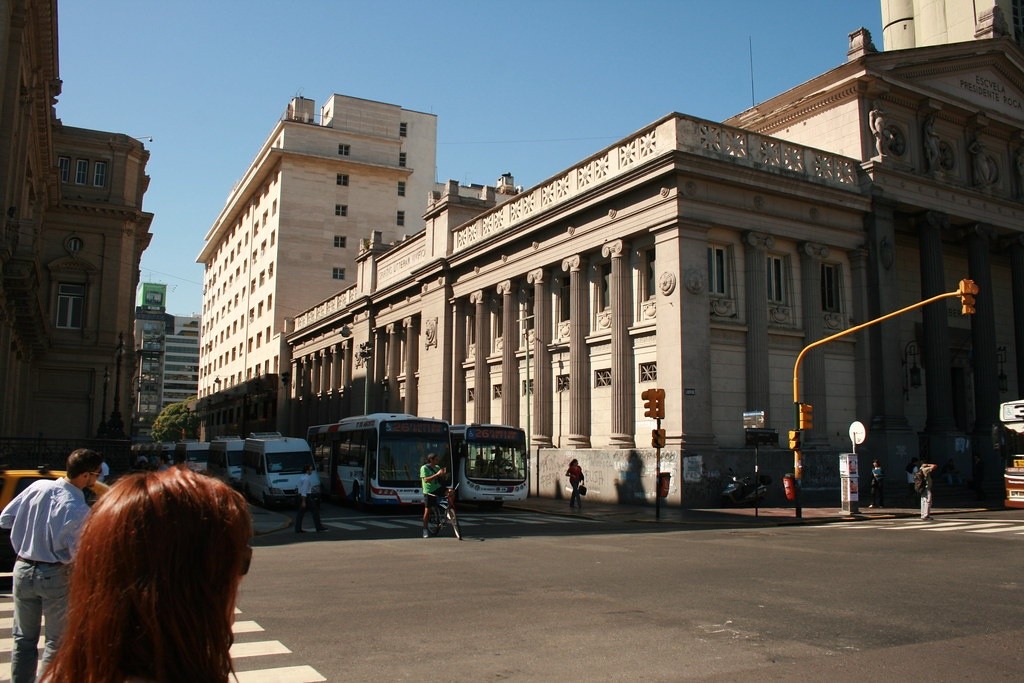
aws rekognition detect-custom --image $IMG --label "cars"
[0,463,111,558]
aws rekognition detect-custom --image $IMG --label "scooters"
[718,467,774,508]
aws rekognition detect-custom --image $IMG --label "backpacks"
[914,469,928,494]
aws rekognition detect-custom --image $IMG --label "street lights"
[96,330,130,440]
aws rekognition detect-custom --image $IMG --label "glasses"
[89,470,103,478]
[234,547,252,576]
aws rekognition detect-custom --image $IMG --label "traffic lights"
[788,431,801,451]
[652,429,665,448]
[960,279,978,314]
[642,389,657,418]
[798,403,813,431]
[656,389,665,420]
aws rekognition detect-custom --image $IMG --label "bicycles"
[426,483,462,540]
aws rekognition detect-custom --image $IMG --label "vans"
[153,432,320,507]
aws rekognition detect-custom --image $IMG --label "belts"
[17,556,63,566]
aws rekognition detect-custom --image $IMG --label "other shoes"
[570,503,575,507]
[422,528,428,539]
[296,528,306,534]
[880,505,885,508]
[318,527,329,533]
[923,516,933,521]
[868,504,875,508]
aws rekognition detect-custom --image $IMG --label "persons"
[129,444,174,473]
[906,459,919,506]
[420,454,454,538]
[920,460,937,522]
[1016,147,1024,196]
[566,459,584,507]
[475,456,511,477]
[925,116,941,171]
[295,467,329,532]
[969,132,988,185]
[0,450,103,683]
[941,457,988,501]
[97,452,110,483]
[869,460,884,508]
[868,100,903,160]
[39,466,250,682]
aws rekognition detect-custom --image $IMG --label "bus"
[991,399,1024,508]
[449,422,530,510]
[307,413,457,510]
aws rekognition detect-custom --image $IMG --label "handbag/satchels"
[579,483,587,495]
[871,479,877,487]
